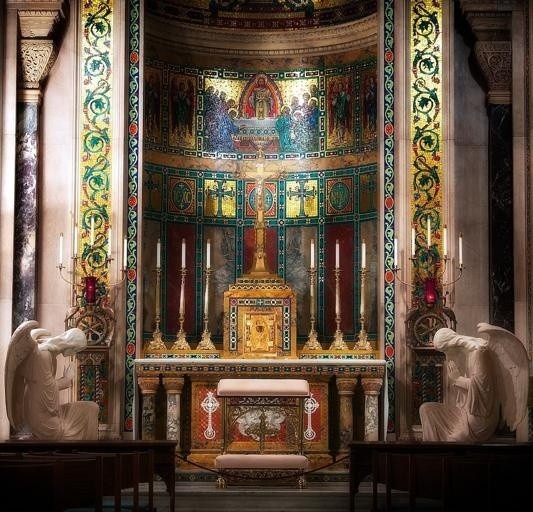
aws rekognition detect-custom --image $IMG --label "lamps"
[56,207,128,306]
[392,213,464,305]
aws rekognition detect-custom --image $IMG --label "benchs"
[214,453,309,490]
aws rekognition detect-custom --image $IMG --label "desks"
[0,438,177,512]
[344,438,533,511]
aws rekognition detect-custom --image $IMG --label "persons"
[418,323,530,443]
[170,78,194,147]
[3,319,100,440]
[362,73,376,132]
[144,74,159,134]
[328,77,353,147]
[274,84,319,151]
[248,77,274,118]
[205,85,240,154]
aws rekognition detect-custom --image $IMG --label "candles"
[155,235,211,270]
[309,237,367,271]
